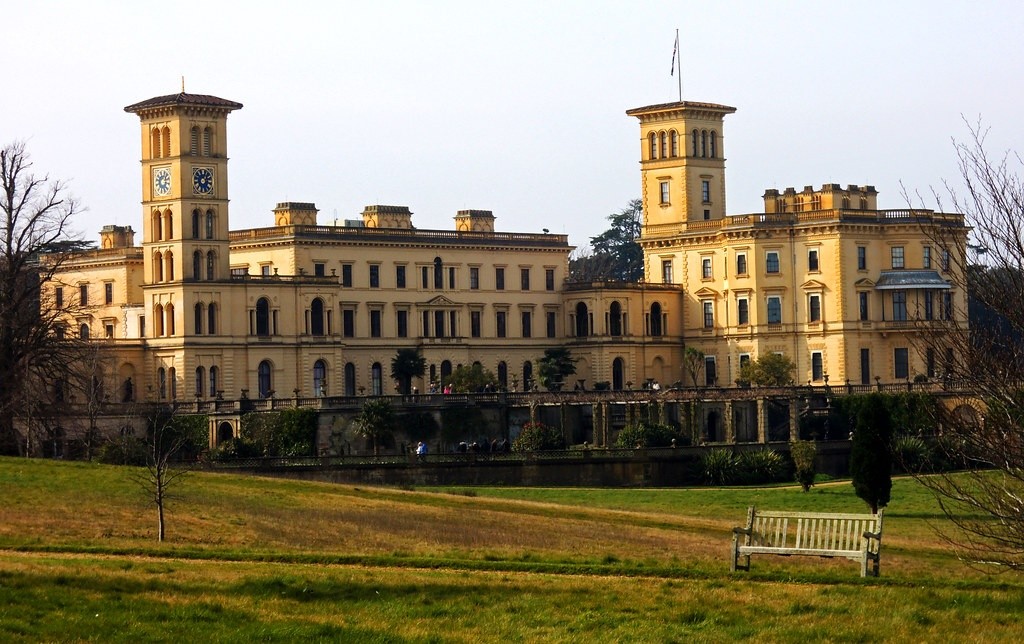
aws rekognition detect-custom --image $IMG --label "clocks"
[191,167,214,195]
[154,168,171,196]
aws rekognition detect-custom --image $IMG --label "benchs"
[730,506,884,579]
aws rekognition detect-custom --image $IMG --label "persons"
[443,384,453,394]
[483,382,496,394]
[413,387,420,403]
[416,442,426,460]
[574,382,579,390]
[652,381,660,391]
[430,384,437,393]
[511,386,516,392]
